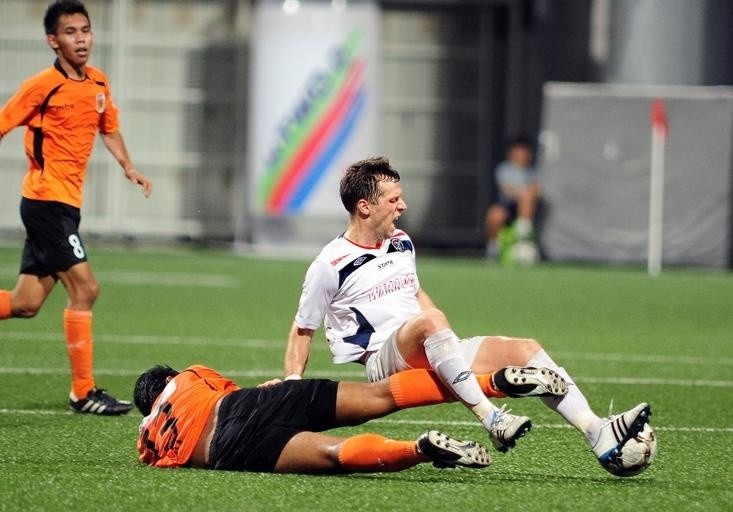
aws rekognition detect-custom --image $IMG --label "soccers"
[603,423,657,476]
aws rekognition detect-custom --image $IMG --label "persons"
[0,1,151,415]
[132,363,568,475]
[482,137,541,260]
[284,155,650,462]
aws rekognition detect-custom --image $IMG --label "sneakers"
[494,366,568,398]
[590,403,653,464]
[489,412,531,454]
[68,386,133,416]
[418,430,493,468]
